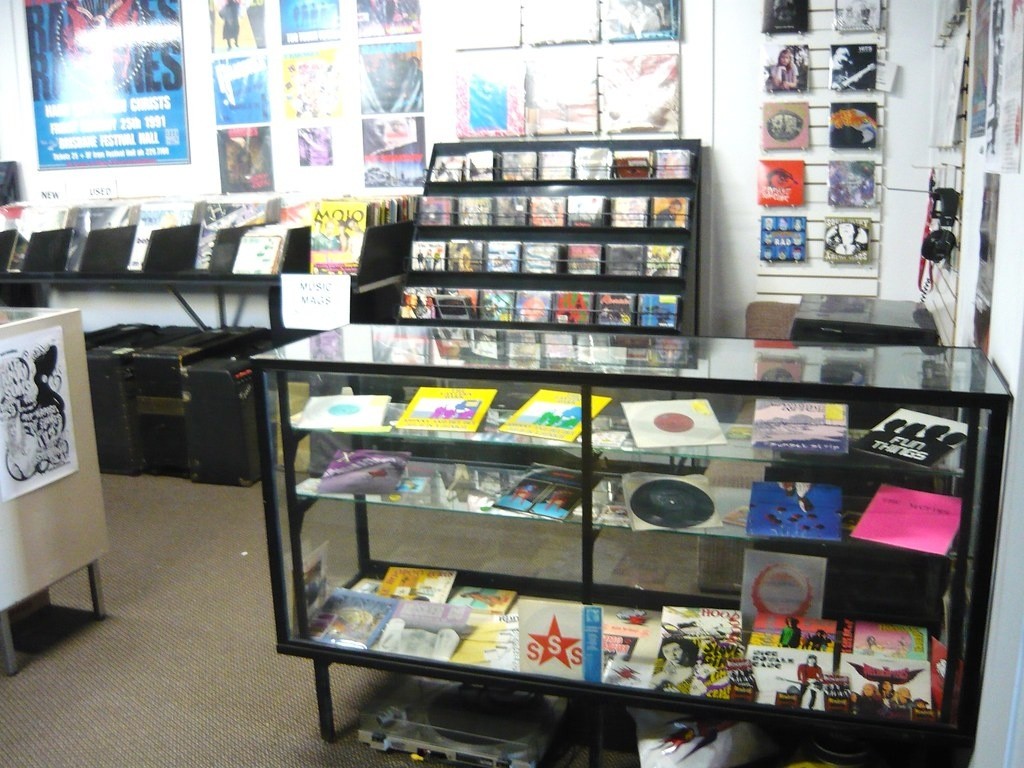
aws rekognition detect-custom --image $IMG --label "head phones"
[921,187,960,262]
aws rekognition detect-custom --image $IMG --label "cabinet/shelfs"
[397,136,705,328]
[250,337,1011,767]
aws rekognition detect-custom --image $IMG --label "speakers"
[82,320,279,488]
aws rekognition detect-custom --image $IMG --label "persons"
[832,48,864,90]
[770,49,799,90]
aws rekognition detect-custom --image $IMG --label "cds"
[629,479,715,529]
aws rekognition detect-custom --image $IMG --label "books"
[196,197,281,270]
[312,194,417,276]
[9,202,206,270]
